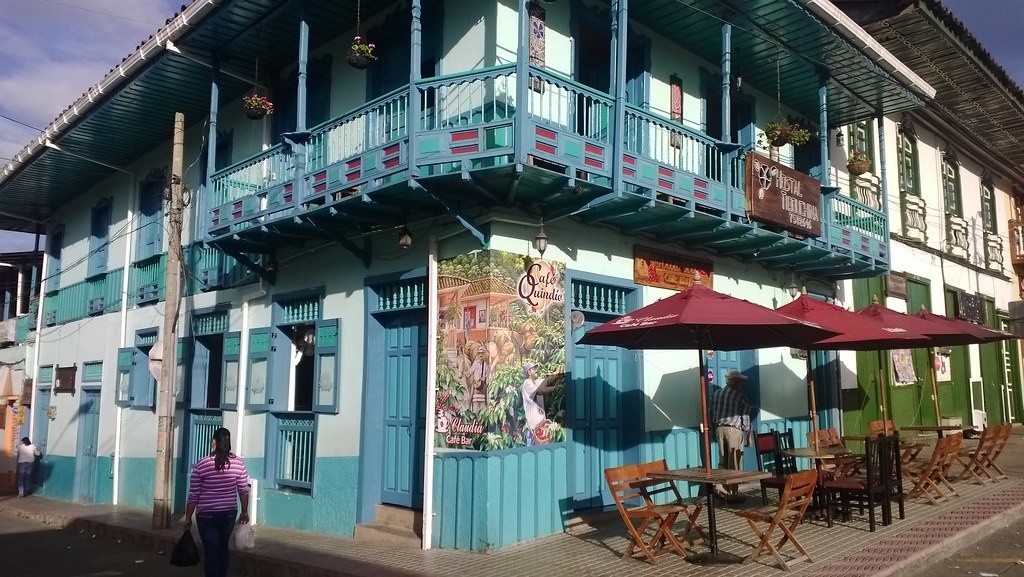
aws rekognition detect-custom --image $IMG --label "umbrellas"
[576,273,844,469]
[774,285,932,451]
[914,304,1021,426]
[854,293,983,433]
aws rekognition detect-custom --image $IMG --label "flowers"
[350,36,378,60]
[849,151,866,163]
[242,94,274,115]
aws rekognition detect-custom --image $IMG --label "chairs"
[604,418,1014,571]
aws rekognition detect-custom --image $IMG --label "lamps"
[836,131,845,148]
[535,217,550,260]
[41,405,57,422]
[398,208,413,250]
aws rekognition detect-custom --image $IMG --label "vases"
[846,161,870,176]
[345,55,373,71]
[246,107,268,120]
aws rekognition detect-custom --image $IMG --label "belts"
[716,424,734,427]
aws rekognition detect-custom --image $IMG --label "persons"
[16,437,41,498]
[712,369,753,498]
[186,428,251,577]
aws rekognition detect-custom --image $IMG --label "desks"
[782,446,853,525]
[842,434,933,443]
[646,467,773,568]
[900,425,979,486]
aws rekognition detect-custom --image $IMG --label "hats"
[726,370,748,380]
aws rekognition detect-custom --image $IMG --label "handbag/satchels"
[229,522,255,550]
[170,530,199,567]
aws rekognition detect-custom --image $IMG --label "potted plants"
[757,119,811,150]
